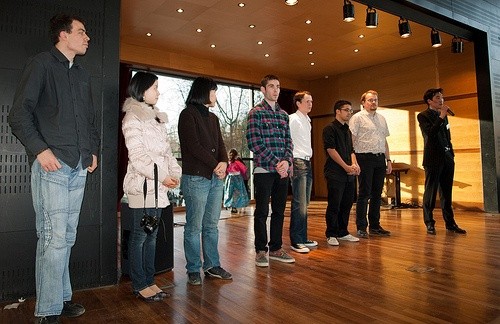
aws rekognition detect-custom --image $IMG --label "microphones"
[447,108,455,116]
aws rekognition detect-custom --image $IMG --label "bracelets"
[387,160,391,162]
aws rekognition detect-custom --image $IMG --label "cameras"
[140,214,160,234]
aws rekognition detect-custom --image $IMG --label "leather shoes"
[448,226,466,234]
[427,225,436,234]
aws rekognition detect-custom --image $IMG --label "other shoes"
[133,288,168,302]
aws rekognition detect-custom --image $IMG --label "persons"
[178,77,232,285]
[225,149,250,212]
[322,100,360,246]
[350,90,392,238]
[121,71,180,304]
[287,90,318,252]
[417,88,466,234]
[8,14,100,324]
[247,74,295,267]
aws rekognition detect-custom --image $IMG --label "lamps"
[398,16,411,38]
[452,37,464,54]
[343,0,355,23]
[366,6,378,28]
[431,27,443,48]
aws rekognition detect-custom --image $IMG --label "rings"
[219,171,222,173]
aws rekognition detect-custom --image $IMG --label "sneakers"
[358,230,368,237]
[269,247,296,262]
[61,302,85,317]
[370,228,390,236]
[186,272,202,285]
[38,315,62,324]
[327,237,339,245]
[290,243,310,252]
[305,240,319,247]
[255,251,269,267]
[337,234,359,242]
[209,266,233,279]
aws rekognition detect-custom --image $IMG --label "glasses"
[339,108,354,113]
